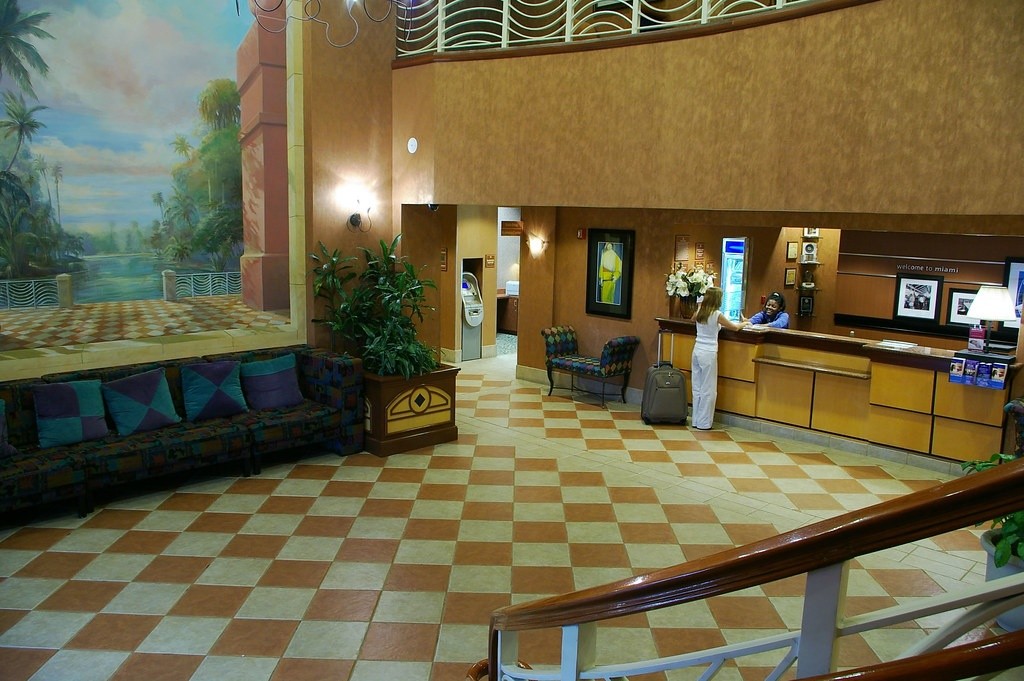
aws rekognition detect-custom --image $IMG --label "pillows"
[29,379,112,448]
[0,397,18,461]
[241,352,306,411]
[176,360,250,422]
[100,367,182,437]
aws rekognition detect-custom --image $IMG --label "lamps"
[954,285,1018,363]
[346,200,372,233]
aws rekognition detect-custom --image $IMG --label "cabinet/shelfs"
[497,295,518,335]
[795,236,824,318]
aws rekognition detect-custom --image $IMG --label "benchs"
[541,325,641,408]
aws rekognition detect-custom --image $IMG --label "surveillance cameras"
[428,204,440,211]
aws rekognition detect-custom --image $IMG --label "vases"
[680,296,696,318]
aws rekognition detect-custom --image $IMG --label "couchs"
[0,342,364,531]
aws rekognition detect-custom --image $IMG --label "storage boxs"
[506,281,519,295]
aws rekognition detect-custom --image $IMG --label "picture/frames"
[695,242,705,260]
[946,287,986,327]
[787,242,798,259]
[784,268,797,286]
[694,261,706,271]
[995,255,1024,332]
[893,272,944,325]
[675,235,691,261]
[584,228,634,320]
[706,261,715,274]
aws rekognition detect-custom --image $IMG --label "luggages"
[641,328,688,425]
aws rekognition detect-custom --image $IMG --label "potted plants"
[959,453,1024,633]
[309,231,461,458]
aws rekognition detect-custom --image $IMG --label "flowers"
[665,264,717,298]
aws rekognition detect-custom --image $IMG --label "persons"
[950,364,962,372]
[743,292,789,329]
[970,339,981,348]
[904,293,925,310]
[692,287,752,430]
